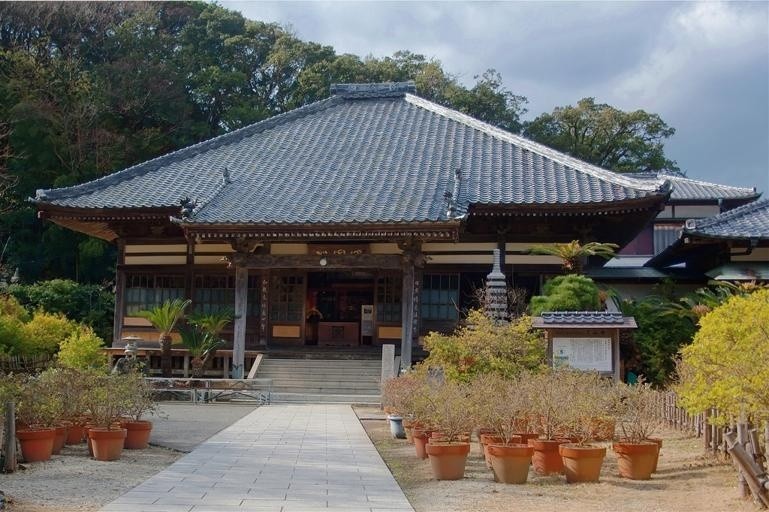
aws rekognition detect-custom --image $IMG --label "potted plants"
[379,306,667,486]
[0,354,171,462]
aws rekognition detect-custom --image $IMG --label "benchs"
[116,376,273,406]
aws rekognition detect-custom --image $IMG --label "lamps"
[319,254,328,267]
